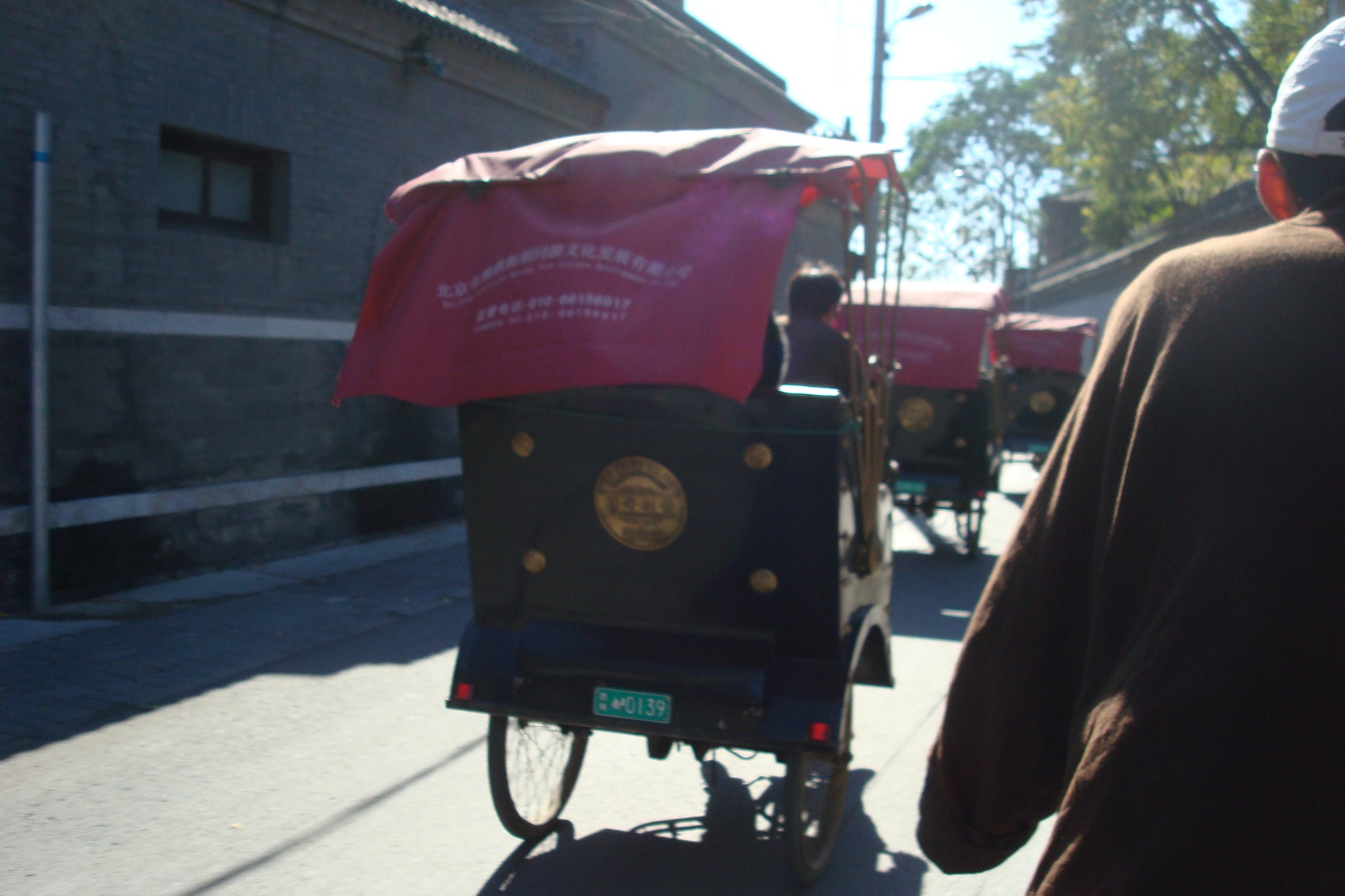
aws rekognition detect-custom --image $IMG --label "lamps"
[408,32,449,87]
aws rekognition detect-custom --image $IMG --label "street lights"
[861,0,939,281]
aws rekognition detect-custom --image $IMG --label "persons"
[914,14,1345,896]
[775,268,866,399]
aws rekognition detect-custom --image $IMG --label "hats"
[1266,16,1345,158]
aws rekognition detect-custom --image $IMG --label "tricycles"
[327,123,907,887]
[976,309,1096,478]
[815,276,1013,561]
